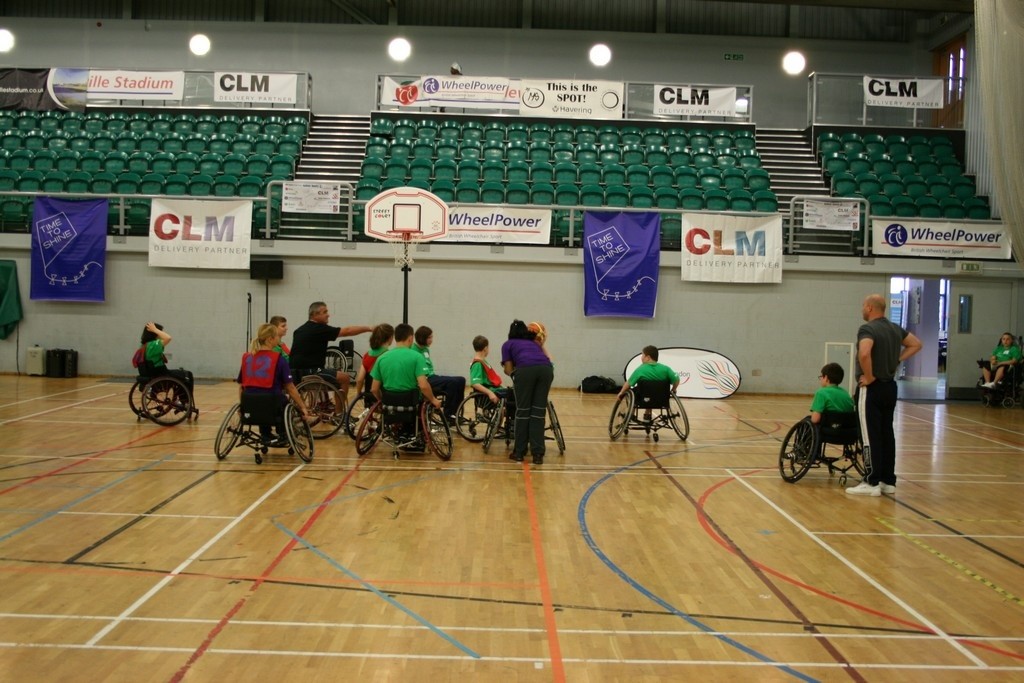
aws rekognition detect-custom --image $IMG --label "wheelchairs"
[454,385,513,444]
[287,368,355,441]
[128,360,200,428]
[213,379,314,464]
[325,338,364,386]
[346,373,383,443]
[482,370,566,455]
[777,414,868,483]
[609,378,690,441]
[425,390,466,427]
[355,389,454,461]
[975,335,1024,409]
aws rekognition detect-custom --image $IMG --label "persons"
[845,294,923,495]
[137,321,194,410]
[981,332,1024,404]
[617,344,680,424]
[783,363,854,459]
[237,301,469,449]
[470,319,555,465]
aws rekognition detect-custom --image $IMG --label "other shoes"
[783,451,795,459]
[510,453,524,461]
[260,433,277,444]
[173,400,188,410]
[643,414,651,420]
[321,401,337,413]
[533,456,543,464]
[332,413,359,423]
[981,381,996,388]
[278,433,290,445]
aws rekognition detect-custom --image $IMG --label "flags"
[583,210,660,319]
[148,198,252,269]
[681,213,783,283]
[30,197,108,302]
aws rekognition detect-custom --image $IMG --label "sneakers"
[846,482,881,496]
[879,481,896,493]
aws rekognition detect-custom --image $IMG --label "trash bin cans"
[46,349,78,378]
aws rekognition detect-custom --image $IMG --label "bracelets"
[898,359,900,364]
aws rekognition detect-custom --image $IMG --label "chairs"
[816,131,992,243]
[353,118,778,250]
[0,108,310,237]
